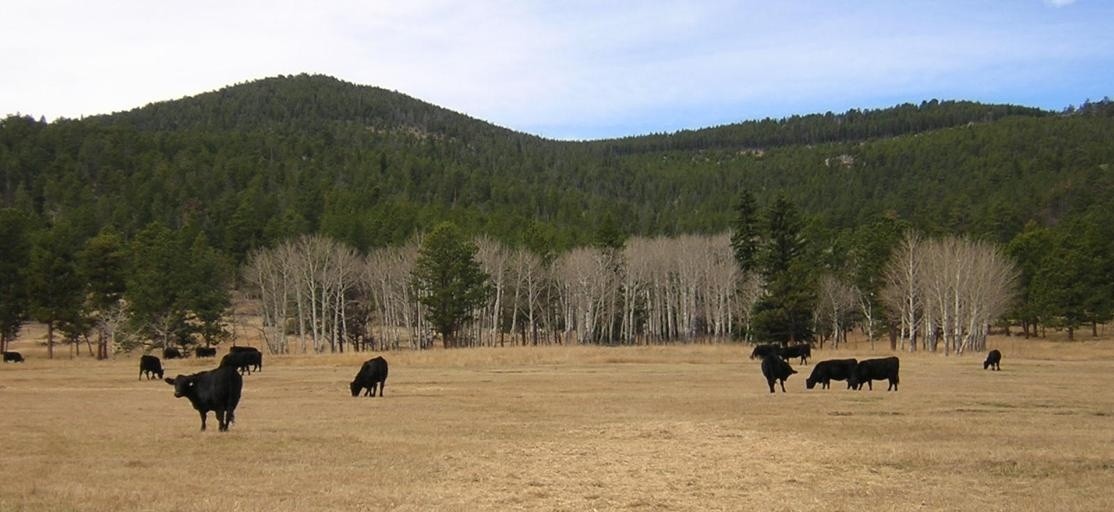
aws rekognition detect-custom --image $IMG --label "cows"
[348,355,390,399]
[805,355,900,393]
[759,354,799,394]
[162,344,264,376]
[2,351,26,364]
[136,354,166,382]
[162,364,243,433]
[749,344,812,366]
[983,349,1002,371]
